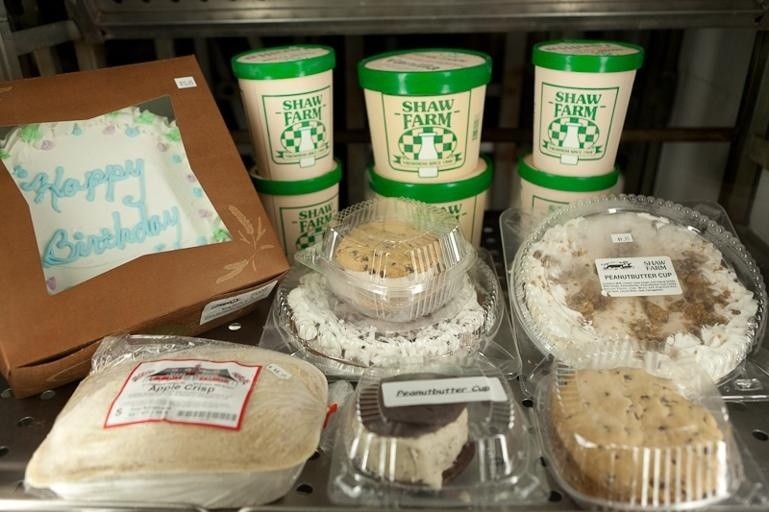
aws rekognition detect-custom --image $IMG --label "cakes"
[345,372,475,491]
[544,366,726,503]
[513,210,756,397]
[287,271,487,368]
[333,220,443,313]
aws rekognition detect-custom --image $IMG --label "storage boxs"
[0,51,291,399]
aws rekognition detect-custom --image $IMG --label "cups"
[531,37,643,175]
[514,153,625,262]
[361,45,485,172]
[237,44,338,179]
[365,163,489,255]
[248,158,342,265]
